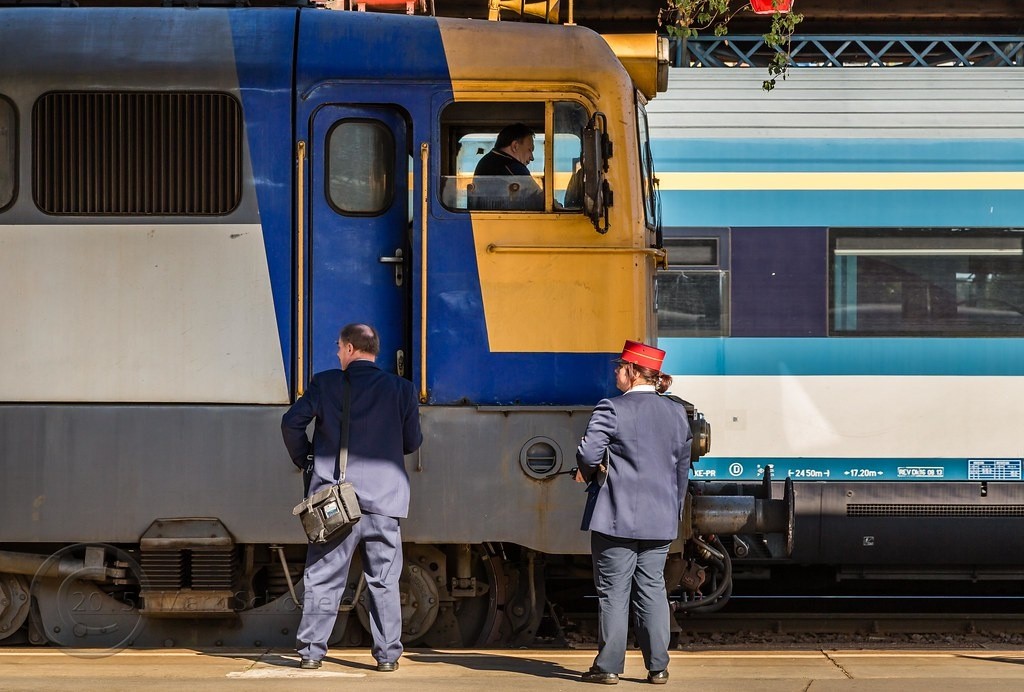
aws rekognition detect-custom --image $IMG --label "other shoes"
[378,661,398,671]
[299,659,321,669]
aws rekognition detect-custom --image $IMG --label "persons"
[576,339,694,685]
[471,122,563,211]
[280,323,424,672]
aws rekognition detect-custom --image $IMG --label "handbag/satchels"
[293,483,362,549]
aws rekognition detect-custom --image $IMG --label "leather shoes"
[647,670,668,683]
[582,665,621,685]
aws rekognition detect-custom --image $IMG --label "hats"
[611,340,666,372]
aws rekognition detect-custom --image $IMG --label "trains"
[1,0,698,654]
[450,58,1024,610]
